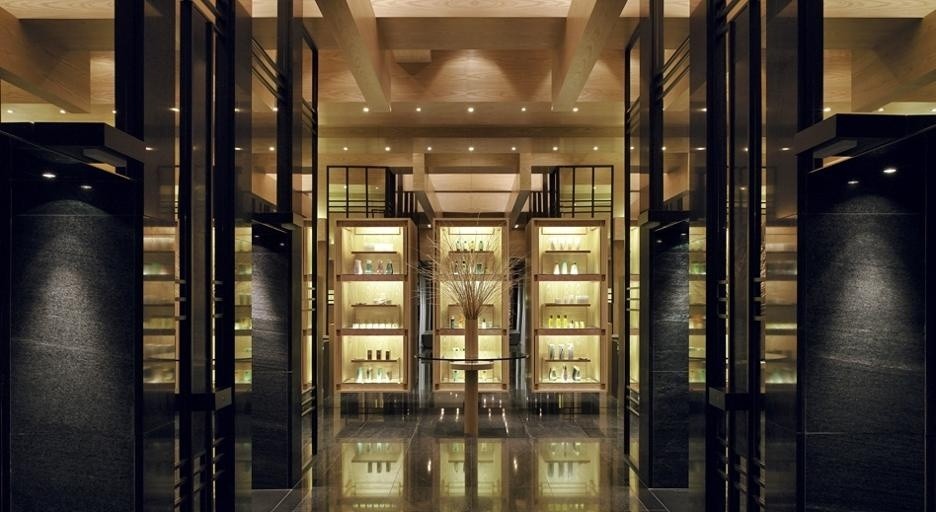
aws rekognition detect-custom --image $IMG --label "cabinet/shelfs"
[529,219,610,395]
[331,218,415,395]
[429,217,512,395]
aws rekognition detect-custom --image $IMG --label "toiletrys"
[553,261,578,274]
[453,371,458,381]
[456,240,483,252]
[482,319,486,328]
[562,365,569,381]
[451,315,455,328]
[548,314,585,329]
[482,371,487,382]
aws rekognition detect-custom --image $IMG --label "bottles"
[453,239,484,274]
[450,315,493,329]
[548,313,586,329]
[354,257,394,276]
[553,261,579,275]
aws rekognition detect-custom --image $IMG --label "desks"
[454,440,494,510]
[450,359,494,439]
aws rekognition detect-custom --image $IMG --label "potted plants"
[393,204,543,359]
